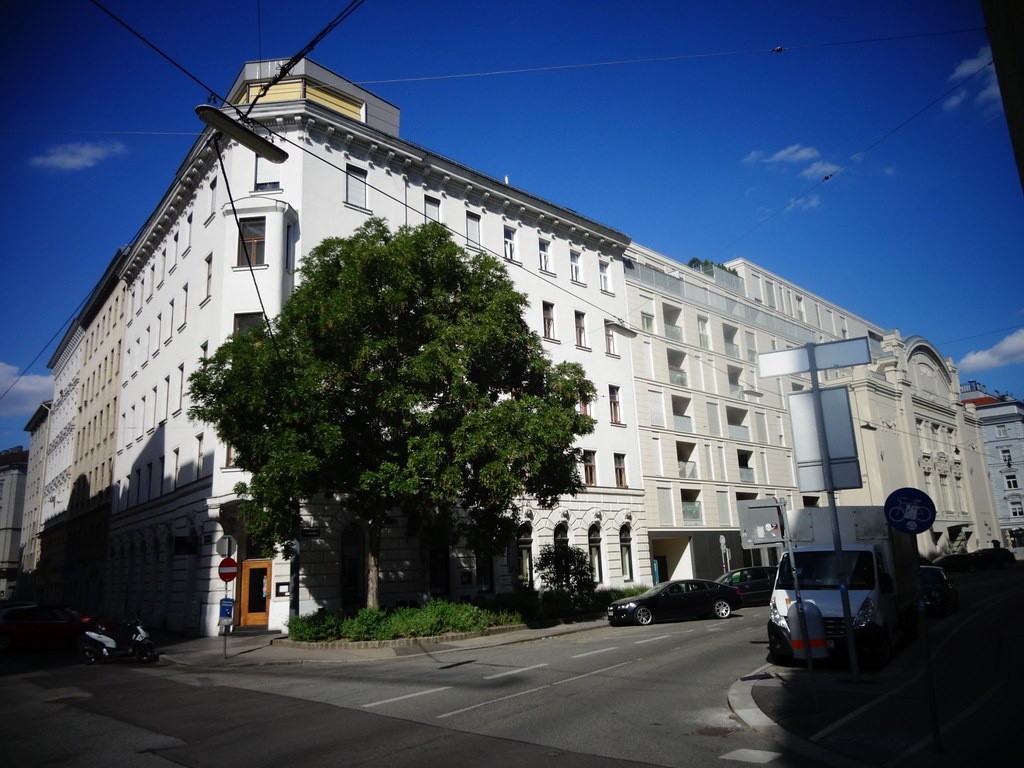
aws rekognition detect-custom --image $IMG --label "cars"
[1,600,116,673]
[607,580,742,626]
[920,548,1016,620]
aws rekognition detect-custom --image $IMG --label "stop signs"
[219,558,238,582]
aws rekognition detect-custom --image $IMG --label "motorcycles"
[83,615,159,663]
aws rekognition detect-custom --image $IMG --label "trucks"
[769,506,921,661]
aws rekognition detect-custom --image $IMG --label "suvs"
[713,566,782,606]
